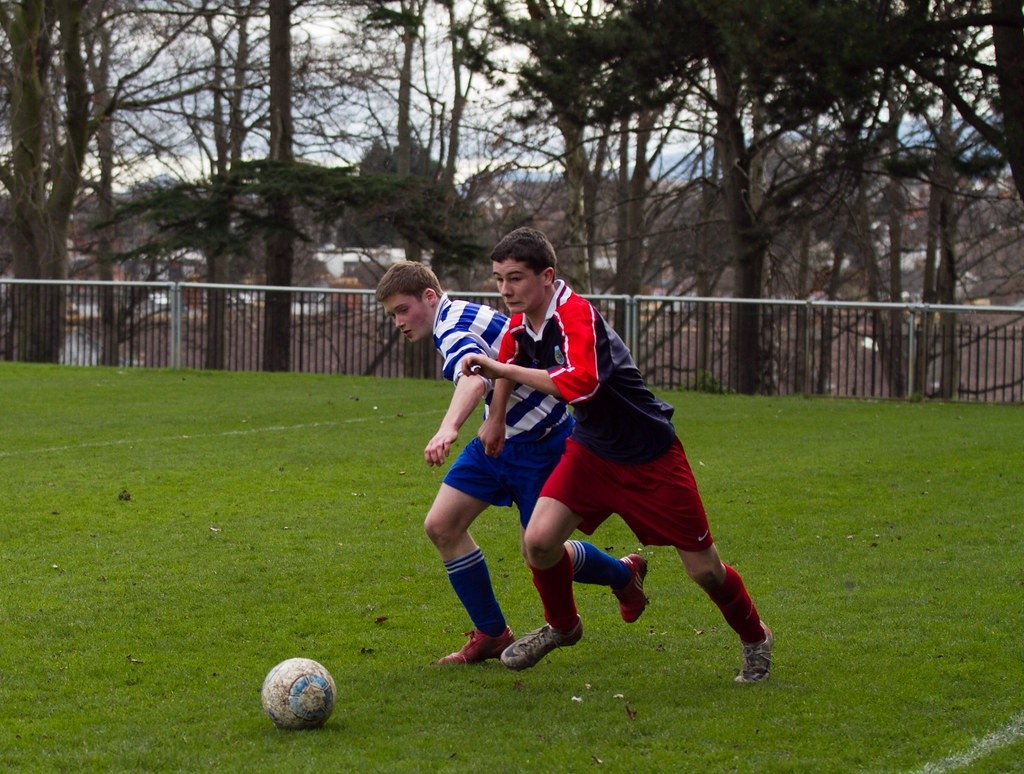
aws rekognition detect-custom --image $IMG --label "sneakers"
[735,619,774,683]
[611,553,648,623]
[436,624,516,664]
[500,613,583,671]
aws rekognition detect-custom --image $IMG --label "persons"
[375,260,649,664]
[462,226,776,686]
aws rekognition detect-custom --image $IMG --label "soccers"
[260,656,337,731]
